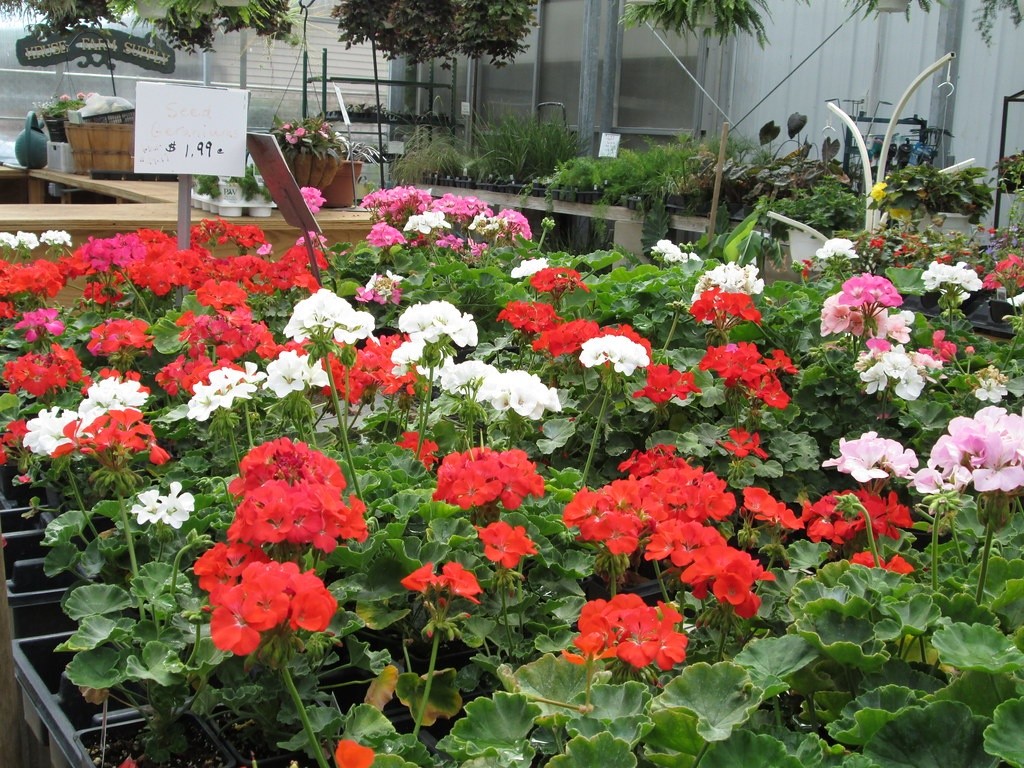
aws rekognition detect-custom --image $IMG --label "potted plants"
[320,132,388,207]
[389,114,998,276]
[190,163,281,216]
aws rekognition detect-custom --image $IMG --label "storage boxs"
[83,109,135,123]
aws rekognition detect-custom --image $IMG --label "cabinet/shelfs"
[994,87,1024,238]
[843,111,927,184]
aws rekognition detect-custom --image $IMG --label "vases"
[41,118,70,143]
[281,148,344,192]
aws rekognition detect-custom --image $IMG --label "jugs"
[16,111,48,168]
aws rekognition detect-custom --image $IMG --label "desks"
[0,161,384,256]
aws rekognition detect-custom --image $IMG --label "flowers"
[36,90,97,118]
[270,113,345,162]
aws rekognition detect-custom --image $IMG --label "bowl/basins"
[64,121,134,173]
